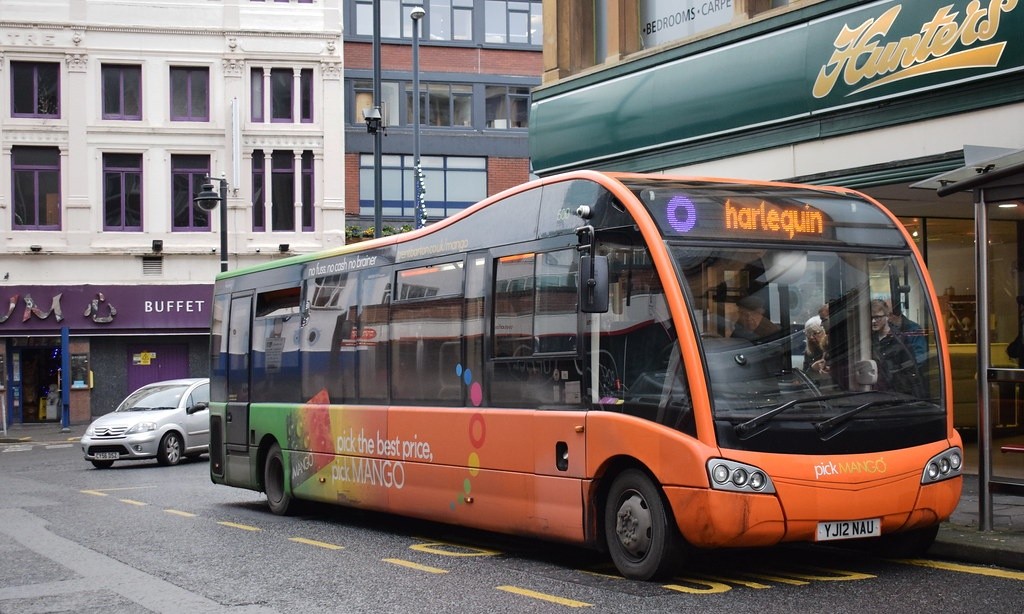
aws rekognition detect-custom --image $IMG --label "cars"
[81,378,209,468]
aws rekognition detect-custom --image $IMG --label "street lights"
[192,176,229,272]
[410,6,425,227]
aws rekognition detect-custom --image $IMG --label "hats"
[736,295,765,310]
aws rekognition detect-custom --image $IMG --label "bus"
[210,168,964,582]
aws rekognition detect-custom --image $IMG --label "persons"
[884,299,926,372]
[728,294,784,377]
[870,297,926,400]
[801,314,831,376]
[817,303,831,376]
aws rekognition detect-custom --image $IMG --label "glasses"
[871,314,886,321]
[806,331,823,339]
